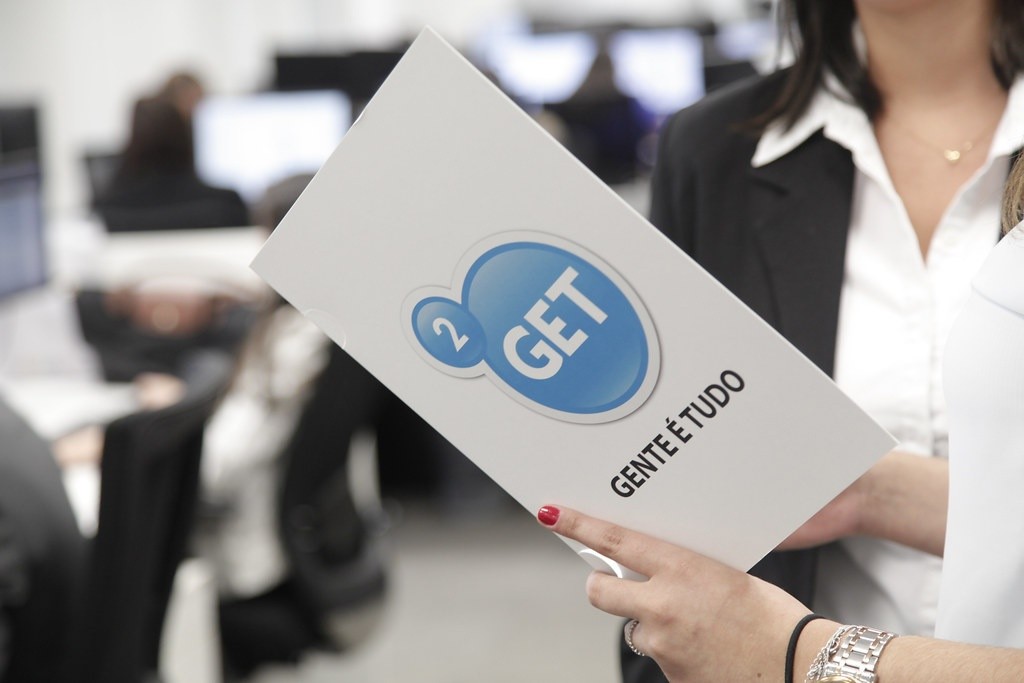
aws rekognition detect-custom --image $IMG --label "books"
[251,22,906,582]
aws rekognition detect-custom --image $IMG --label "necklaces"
[884,105,998,163]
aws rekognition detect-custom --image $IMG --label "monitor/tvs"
[0,102,48,298]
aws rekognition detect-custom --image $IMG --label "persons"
[0,24,758,682]
[534,152,1023,683]
[610,2,1023,682]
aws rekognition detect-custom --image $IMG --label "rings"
[626,620,646,658]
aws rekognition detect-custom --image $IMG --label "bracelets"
[782,611,858,683]
[816,624,901,683]
